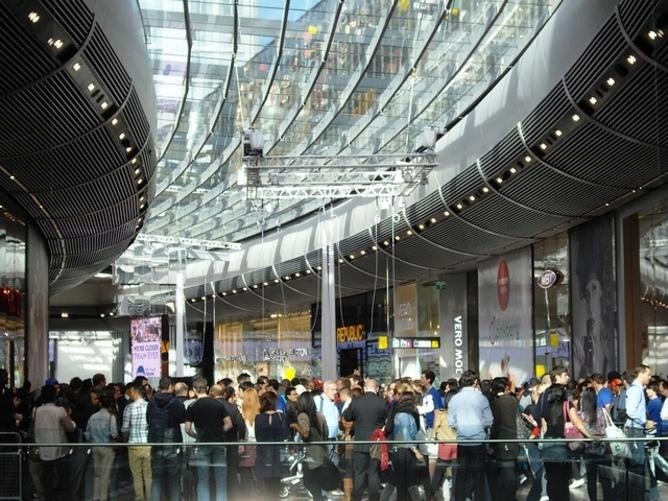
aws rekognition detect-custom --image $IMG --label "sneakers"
[569,478,584,490]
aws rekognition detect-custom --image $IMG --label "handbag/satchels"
[26,443,39,462]
[602,408,633,458]
[369,442,381,459]
[563,401,585,451]
[318,456,340,491]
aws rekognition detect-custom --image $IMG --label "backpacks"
[612,384,642,423]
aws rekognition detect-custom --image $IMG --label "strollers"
[276,452,313,499]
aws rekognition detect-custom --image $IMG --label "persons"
[569,223,612,378]
[0,365,665,498]
